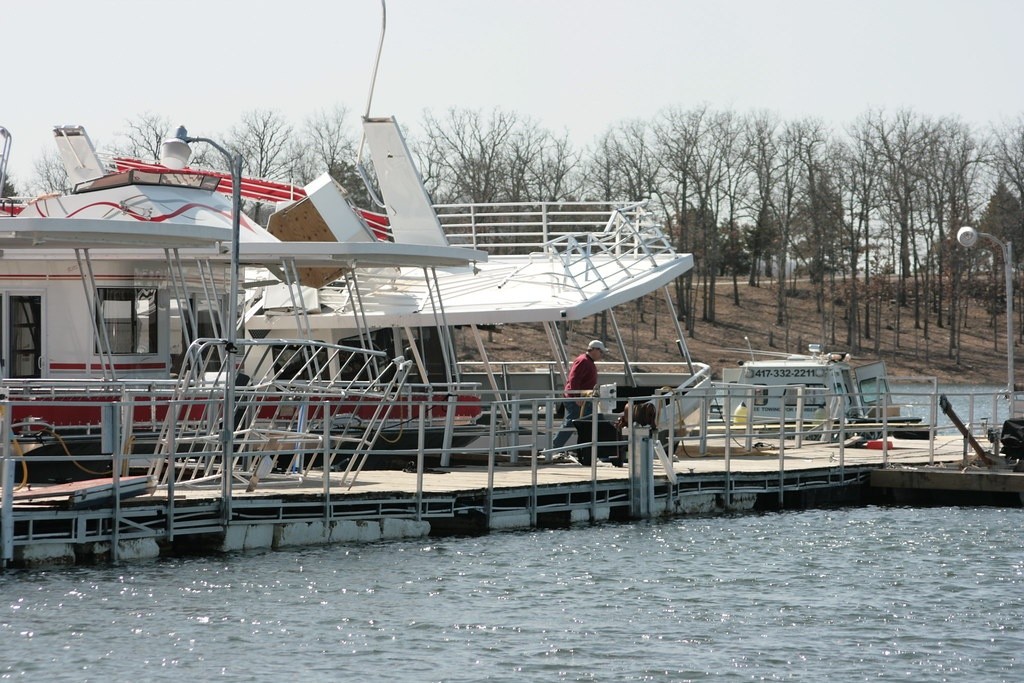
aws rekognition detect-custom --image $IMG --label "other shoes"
[596,460,605,467]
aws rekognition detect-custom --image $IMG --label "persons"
[553,340,608,446]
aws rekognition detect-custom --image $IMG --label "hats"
[589,340,608,352]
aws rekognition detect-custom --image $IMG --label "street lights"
[957,225,1014,414]
[157,125,242,523]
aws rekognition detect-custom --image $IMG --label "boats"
[0,0,715,481]
[686,339,935,443]
